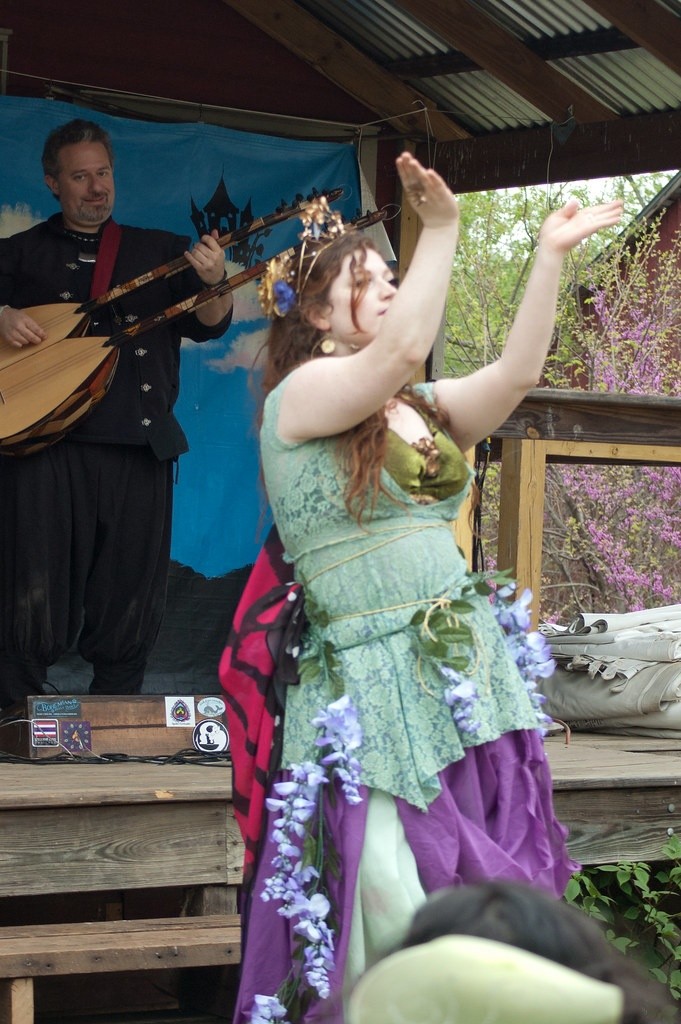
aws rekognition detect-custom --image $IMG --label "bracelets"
[0,305,10,313]
[201,270,228,288]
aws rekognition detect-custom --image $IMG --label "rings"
[407,184,426,206]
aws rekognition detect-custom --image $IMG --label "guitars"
[1,182,399,455]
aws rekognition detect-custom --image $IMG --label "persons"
[233,152,623,1024]
[0,120,233,757]
[380,878,681,1024]
